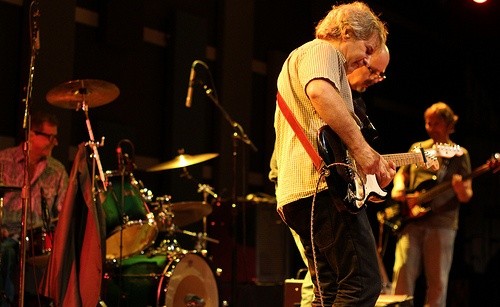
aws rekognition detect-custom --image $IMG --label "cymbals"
[46,80,120,108]
[150,201,212,231]
[138,152,220,171]
[0,182,22,192]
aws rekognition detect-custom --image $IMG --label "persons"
[0,113,69,240]
[391,102,472,307]
[269,1,397,307]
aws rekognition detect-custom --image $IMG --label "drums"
[94,169,159,260]
[99,245,220,307]
[7,225,56,271]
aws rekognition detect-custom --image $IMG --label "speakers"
[237,198,291,284]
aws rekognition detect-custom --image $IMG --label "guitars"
[316,124,439,216]
[377,153,500,230]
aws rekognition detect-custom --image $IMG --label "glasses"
[34,131,58,143]
[365,64,386,81]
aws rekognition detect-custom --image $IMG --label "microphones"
[186,66,195,107]
[32,7,40,50]
